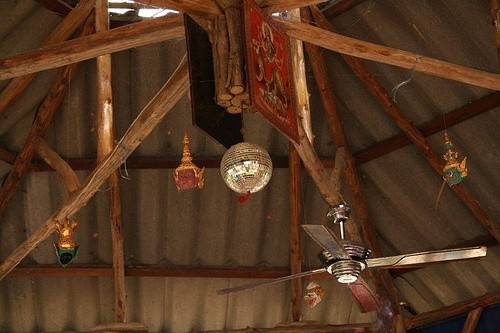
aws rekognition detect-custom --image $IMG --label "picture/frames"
[242,0,300,147]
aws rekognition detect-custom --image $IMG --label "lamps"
[220,101,272,202]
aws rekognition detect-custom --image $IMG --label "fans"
[215,204,486,314]
